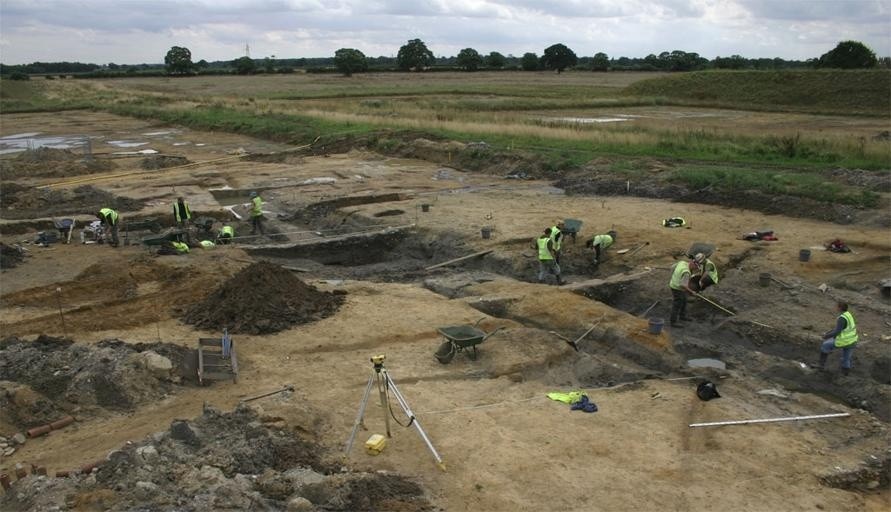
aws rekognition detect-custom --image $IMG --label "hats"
[695,253,706,264]
[249,192,256,197]
[559,221,565,225]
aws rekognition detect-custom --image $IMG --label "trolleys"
[439,316,504,362]
[562,218,584,244]
[690,243,716,263]
[52,219,79,241]
[198,337,240,389]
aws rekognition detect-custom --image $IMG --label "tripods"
[342,363,447,473]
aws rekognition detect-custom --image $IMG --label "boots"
[812,352,827,369]
[844,367,849,376]
[556,275,566,285]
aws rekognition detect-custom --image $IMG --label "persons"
[689,253,718,290]
[669,258,699,328]
[174,196,191,225]
[221,226,233,244]
[587,234,613,263]
[550,221,565,273]
[95,208,119,247]
[245,192,263,233]
[811,298,858,376]
[536,228,567,285]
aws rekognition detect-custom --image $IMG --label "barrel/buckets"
[758,271,772,288]
[480,225,494,240]
[434,338,456,365]
[647,317,666,336]
[421,202,429,212]
[365,434,387,456]
[799,248,812,263]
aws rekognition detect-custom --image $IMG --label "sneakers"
[672,323,684,327]
[680,317,691,321]
